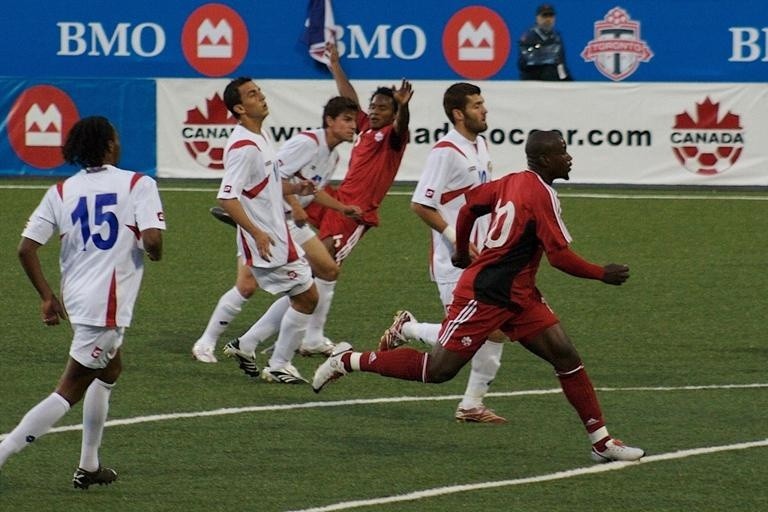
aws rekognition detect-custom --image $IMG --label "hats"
[539,4,555,17]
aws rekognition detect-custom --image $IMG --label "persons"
[375,79,512,426]
[216,74,320,389]
[298,46,415,289]
[514,3,571,83]
[0,116,170,491]
[311,129,648,466]
[188,97,361,363]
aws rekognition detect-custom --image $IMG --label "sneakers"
[298,338,337,357]
[191,344,218,364]
[377,308,416,354]
[454,406,507,425]
[311,340,352,392]
[260,361,310,385]
[72,465,118,488]
[223,338,260,378]
[588,440,645,463]
[211,204,238,227]
